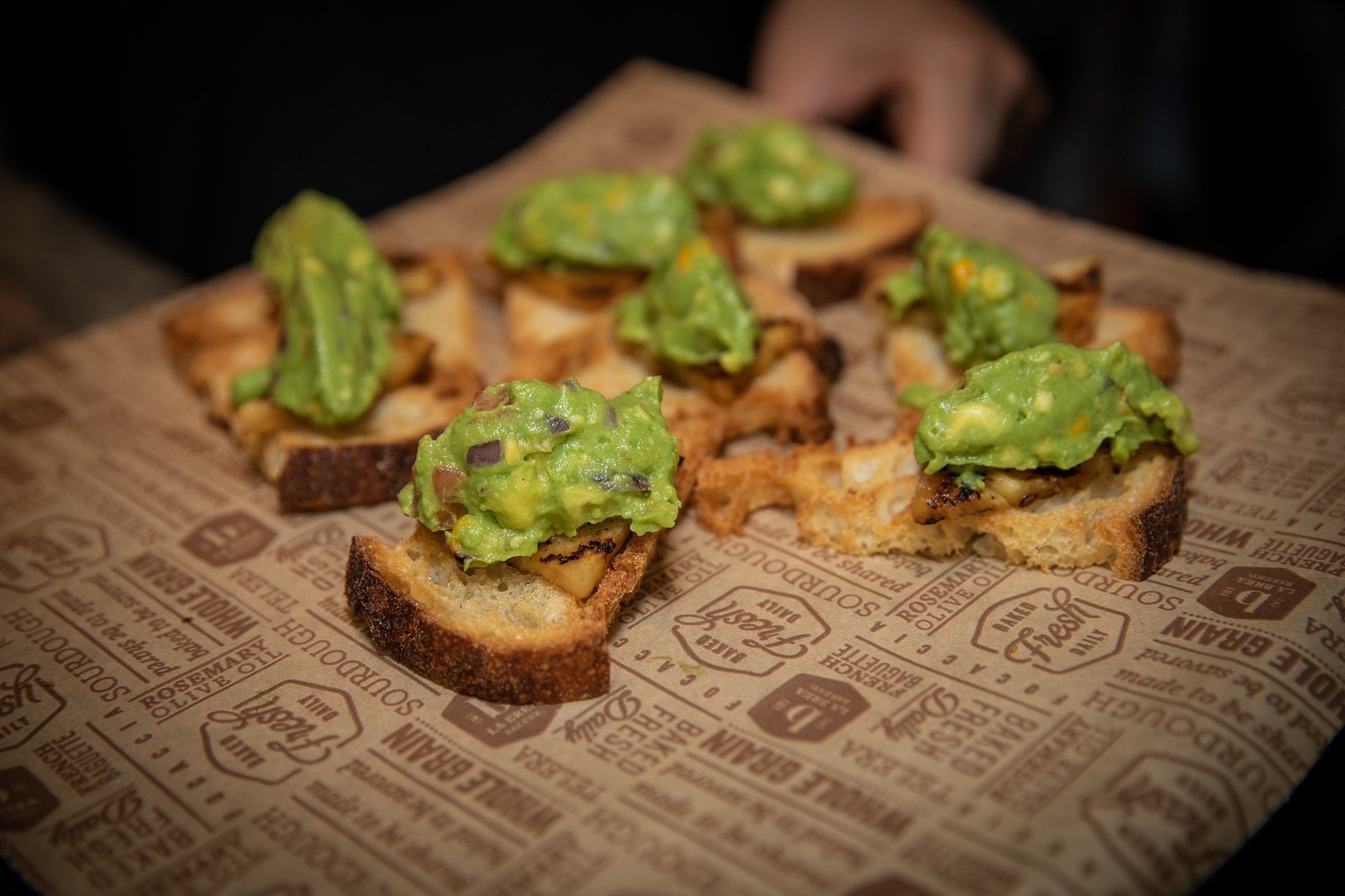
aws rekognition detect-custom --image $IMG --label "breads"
[157,113,1197,704]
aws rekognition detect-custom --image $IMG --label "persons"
[0,0,1196,343]
